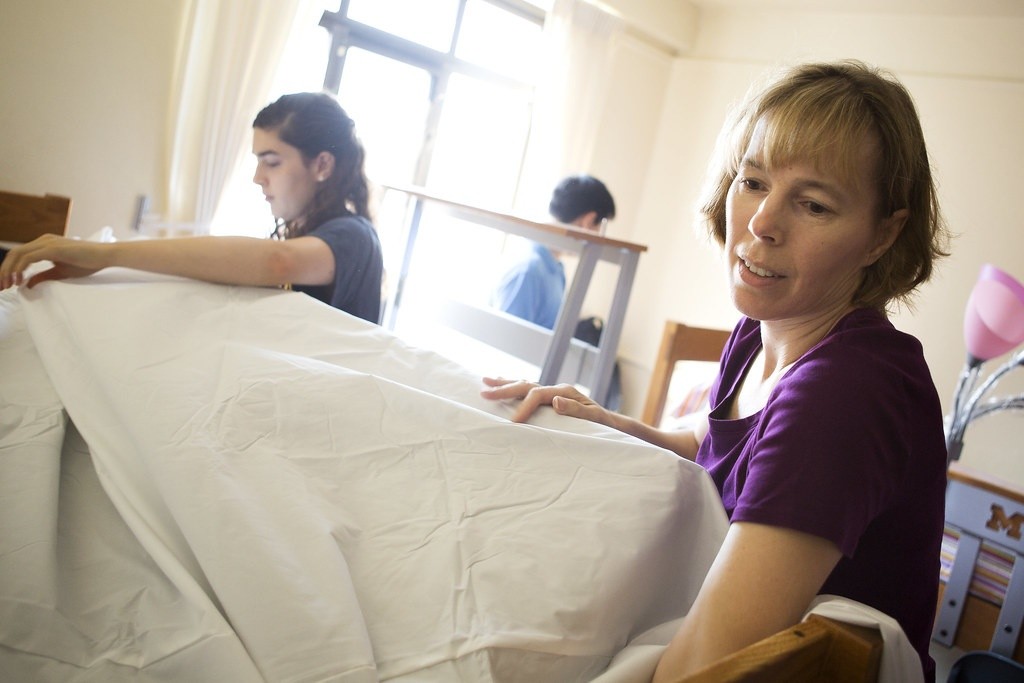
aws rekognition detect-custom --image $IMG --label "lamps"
[946,261,1024,467]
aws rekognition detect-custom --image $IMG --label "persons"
[494,175,615,330]
[481,61,949,683]
[0,92,384,324]
